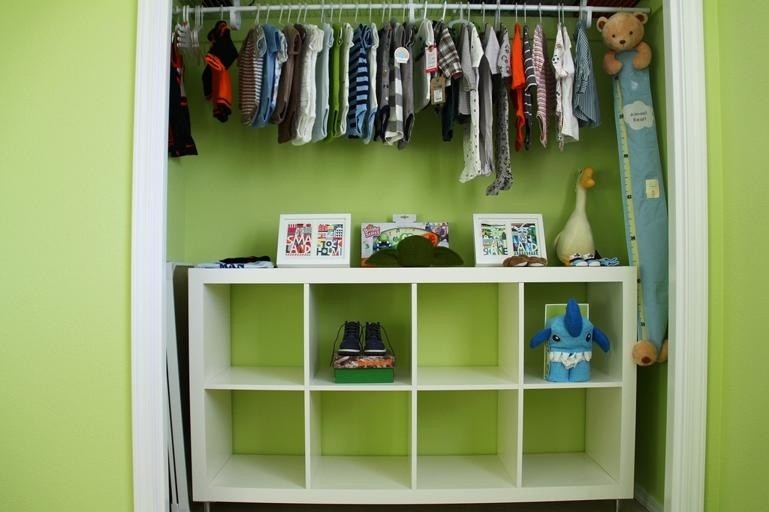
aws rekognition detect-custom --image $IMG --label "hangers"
[354,0,591,31]
[169,0,355,50]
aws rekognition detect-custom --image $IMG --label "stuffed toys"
[551,166,597,266]
[363,235,464,269]
[503,254,547,267]
[530,298,610,382]
[596,12,669,366]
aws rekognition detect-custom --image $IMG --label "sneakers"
[365,322,387,355]
[339,321,360,356]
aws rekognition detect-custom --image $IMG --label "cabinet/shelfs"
[182,257,642,506]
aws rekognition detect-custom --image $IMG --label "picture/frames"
[275,212,354,269]
[473,213,549,266]
